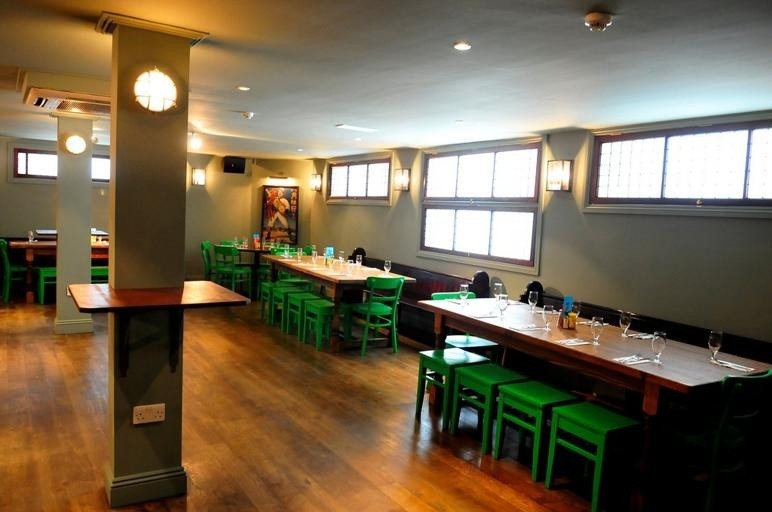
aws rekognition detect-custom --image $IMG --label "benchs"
[343,255,477,348]
[525,290,771,420]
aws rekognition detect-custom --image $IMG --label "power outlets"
[134,403,167,425]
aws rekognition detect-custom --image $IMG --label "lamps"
[64,135,88,154]
[545,159,571,192]
[392,169,410,192]
[191,167,206,187]
[119,59,189,118]
[309,173,320,191]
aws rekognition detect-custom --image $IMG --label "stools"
[36,265,57,305]
[416,348,641,512]
[261,281,335,353]
[89,266,109,284]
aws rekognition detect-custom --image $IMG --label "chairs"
[220,239,245,289]
[214,245,253,295]
[354,277,405,358]
[199,239,233,281]
[0,238,28,305]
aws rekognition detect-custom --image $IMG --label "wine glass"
[312,251,317,264]
[650,331,666,364]
[619,312,632,336]
[356,255,362,267]
[312,245,317,251]
[384,261,391,274]
[339,251,344,260]
[497,295,508,316]
[323,248,326,256]
[284,244,289,258]
[493,283,503,302]
[528,291,538,313]
[543,305,553,331]
[591,317,603,346]
[460,285,468,304]
[348,260,353,274]
[707,331,723,361]
[297,247,302,265]
[572,300,581,320]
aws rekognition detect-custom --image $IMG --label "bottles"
[557,309,563,328]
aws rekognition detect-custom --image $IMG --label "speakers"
[223,156,246,174]
